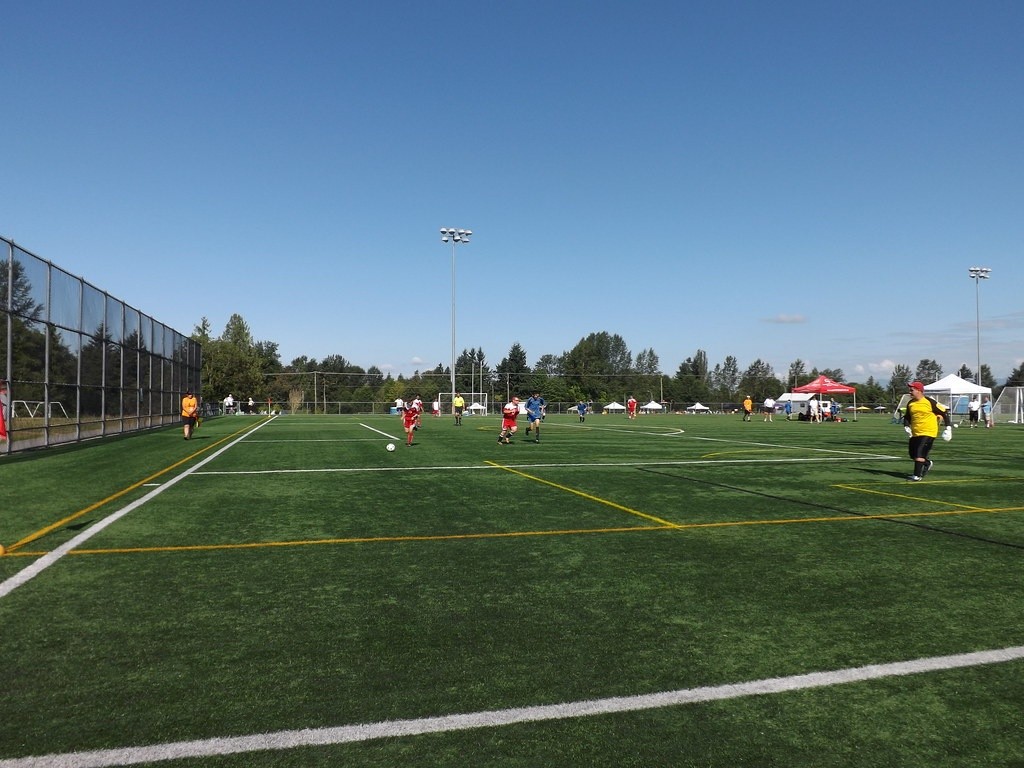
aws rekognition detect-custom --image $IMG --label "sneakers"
[922,461,933,475]
[913,475,923,481]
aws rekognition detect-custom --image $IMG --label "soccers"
[386,443,396,452]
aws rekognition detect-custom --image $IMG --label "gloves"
[942,425,952,441]
[904,426,912,438]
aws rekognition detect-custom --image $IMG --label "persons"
[497,397,520,445]
[453,393,464,425]
[394,395,424,447]
[785,399,792,421]
[524,390,545,443]
[968,396,992,427]
[809,397,821,423]
[743,395,753,422]
[181,393,197,440]
[897,383,952,483]
[248,398,254,413]
[577,401,587,421]
[626,395,638,418]
[432,399,439,416]
[829,398,837,420]
[225,394,234,410]
[764,395,775,422]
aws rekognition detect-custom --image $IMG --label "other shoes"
[743,418,745,421]
[504,437,509,443]
[974,425,977,427]
[526,428,529,436]
[770,419,772,422]
[986,424,990,428]
[408,442,411,446]
[764,420,767,422]
[497,441,502,446]
[970,424,973,428]
[535,438,539,443]
[184,437,189,440]
[748,420,751,422]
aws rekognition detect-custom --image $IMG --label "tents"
[687,402,709,413]
[604,402,625,414]
[639,401,662,413]
[924,374,993,425]
[792,375,856,421]
[467,402,486,414]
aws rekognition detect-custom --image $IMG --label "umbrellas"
[846,406,886,413]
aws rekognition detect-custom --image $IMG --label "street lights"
[968,267,992,420]
[440,228,472,414]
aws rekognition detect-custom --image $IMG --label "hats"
[747,395,750,398]
[908,382,924,392]
[187,391,192,395]
